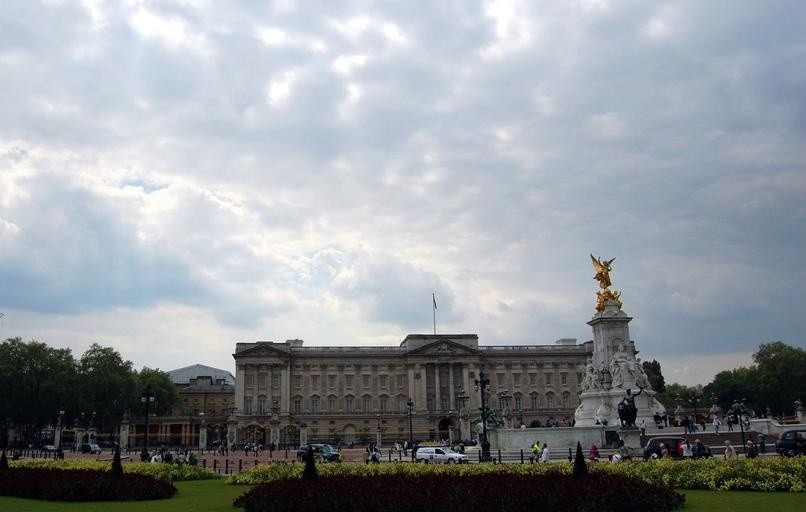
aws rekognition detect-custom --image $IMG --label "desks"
[519,408,523,423]
[673,389,747,423]
[448,410,455,444]
[375,413,382,446]
[58,407,65,449]
[140,385,156,455]
[80,406,98,444]
[405,394,417,451]
[473,369,493,464]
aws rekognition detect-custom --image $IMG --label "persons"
[365,439,382,464]
[538,442,551,463]
[530,440,542,462]
[243,439,264,457]
[440,434,477,456]
[588,440,633,465]
[595,288,623,311]
[590,254,616,289]
[337,438,354,461]
[482,404,499,425]
[794,431,805,458]
[391,439,418,457]
[616,387,642,425]
[519,416,574,429]
[641,396,783,461]
[142,444,198,466]
[215,441,237,456]
[580,343,652,392]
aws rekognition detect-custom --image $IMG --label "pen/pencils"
[79,443,103,454]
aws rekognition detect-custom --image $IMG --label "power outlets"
[416,448,471,466]
[294,443,344,464]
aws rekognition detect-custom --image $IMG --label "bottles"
[660,443,664,448]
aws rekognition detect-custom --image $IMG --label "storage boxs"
[609,453,645,462]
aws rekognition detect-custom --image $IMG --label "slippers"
[531,443,535,448]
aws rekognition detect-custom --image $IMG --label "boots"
[774,428,806,457]
[98,440,112,448]
[642,435,711,465]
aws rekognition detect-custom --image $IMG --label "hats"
[41,444,58,452]
